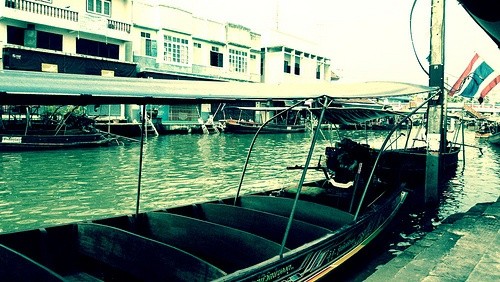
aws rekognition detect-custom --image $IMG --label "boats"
[219,100,315,132]
[0,92,120,148]
[309,107,468,180]
[468,109,500,143]
[0,68,444,282]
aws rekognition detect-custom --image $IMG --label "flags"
[447,53,500,105]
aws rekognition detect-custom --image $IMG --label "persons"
[482,123,500,134]
